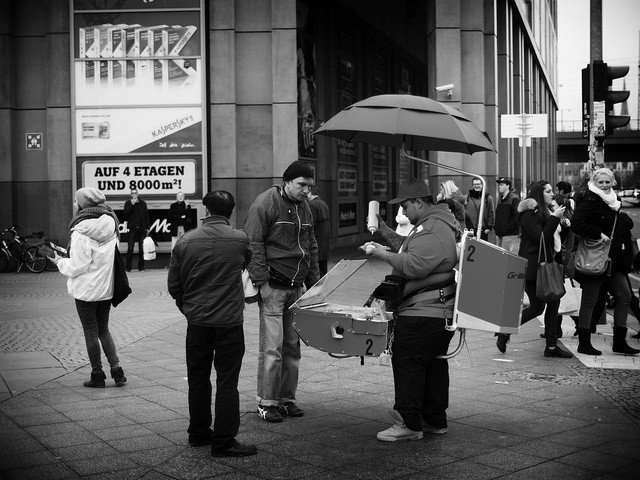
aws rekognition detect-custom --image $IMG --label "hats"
[283,161,314,182]
[388,180,431,204]
[76,187,106,209]
[496,177,511,184]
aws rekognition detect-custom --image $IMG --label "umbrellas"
[314,94,498,156]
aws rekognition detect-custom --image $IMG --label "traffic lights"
[582,61,630,143]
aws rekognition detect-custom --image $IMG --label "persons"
[169,192,192,267]
[494,178,522,255]
[366,179,459,443]
[436,179,466,241]
[571,168,640,355]
[46,186,127,388]
[124,190,149,271]
[464,178,494,242]
[553,182,573,202]
[494,180,573,357]
[307,184,331,289]
[242,161,321,422]
[168,190,257,456]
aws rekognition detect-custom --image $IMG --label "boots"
[84,371,106,387]
[578,329,602,355]
[111,367,127,385]
[613,327,640,354]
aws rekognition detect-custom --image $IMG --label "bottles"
[368,199,379,236]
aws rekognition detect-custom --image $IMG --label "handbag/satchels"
[143,233,156,261]
[241,269,260,304]
[112,243,132,307]
[373,275,406,301]
[574,212,618,276]
[536,232,566,302]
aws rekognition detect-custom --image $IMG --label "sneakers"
[497,333,510,353]
[278,402,303,416]
[544,346,573,358]
[376,410,423,442]
[257,405,283,422]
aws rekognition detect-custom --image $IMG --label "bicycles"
[1,224,65,274]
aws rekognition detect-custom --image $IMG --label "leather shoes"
[212,443,257,456]
